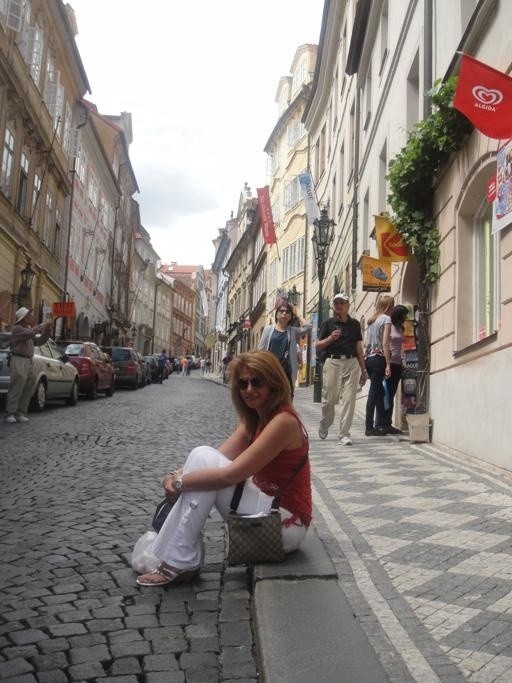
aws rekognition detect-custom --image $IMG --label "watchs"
[173,476,183,495]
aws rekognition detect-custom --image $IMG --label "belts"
[326,354,355,359]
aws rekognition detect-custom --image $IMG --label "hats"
[332,294,349,301]
[16,306,32,323]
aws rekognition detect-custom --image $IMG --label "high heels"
[135,562,200,587]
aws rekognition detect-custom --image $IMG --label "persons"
[386,305,409,434]
[174,356,212,376]
[256,302,313,404]
[222,351,232,384]
[155,350,166,385]
[5,305,53,423]
[135,349,311,586]
[363,295,395,436]
[314,293,367,446]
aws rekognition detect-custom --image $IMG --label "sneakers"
[318,425,328,439]
[7,415,30,423]
[366,426,400,436]
[337,435,352,446]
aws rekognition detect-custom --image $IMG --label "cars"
[99,345,143,388]
[145,351,206,383]
[55,339,118,398]
[0,332,82,412]
[137,351,153,386]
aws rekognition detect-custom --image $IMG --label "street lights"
[288,282,300,307]
[310,201,337,402]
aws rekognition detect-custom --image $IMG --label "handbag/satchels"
[222,513,286,567]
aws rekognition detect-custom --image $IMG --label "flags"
[452,53,512,139]
[256,188,276,245]
[299,173,320,223]
[374,215,412,262]
[361,256,392,292]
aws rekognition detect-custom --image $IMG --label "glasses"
[239,378,264,389]
[278,308,292,313]
